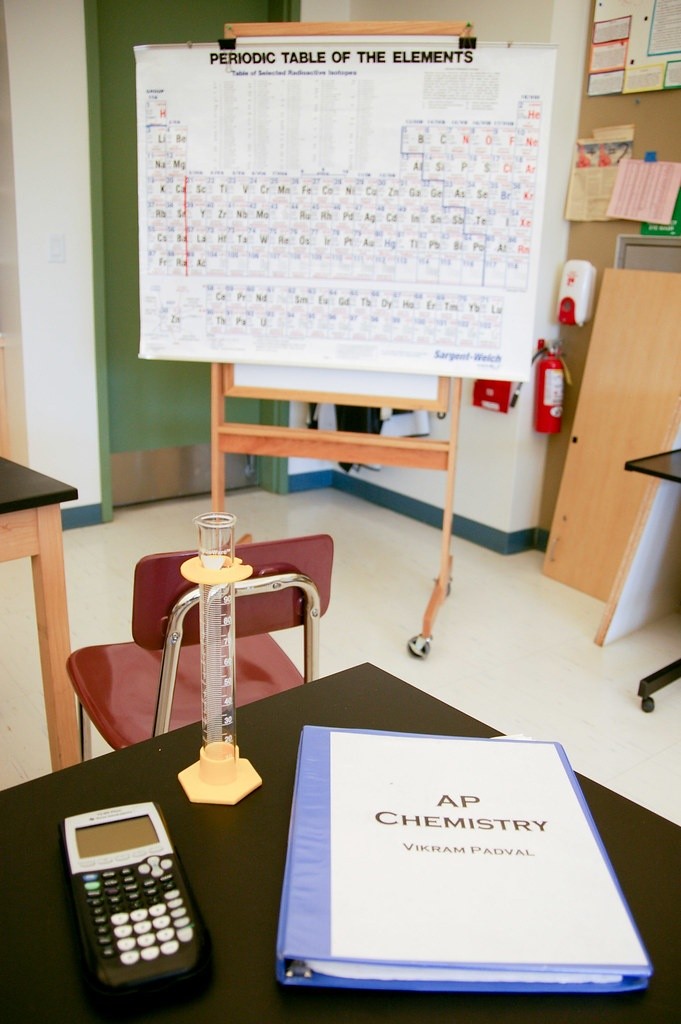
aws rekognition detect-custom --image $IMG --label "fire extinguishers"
[510,341,569,434]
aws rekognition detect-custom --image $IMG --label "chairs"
[66,533,334,765]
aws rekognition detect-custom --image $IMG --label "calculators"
[58,800,216,993]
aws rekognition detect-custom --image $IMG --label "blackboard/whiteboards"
[211,21,474,414]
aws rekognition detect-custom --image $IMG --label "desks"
[0,457,83,775]
[624,448,681,713]
[0,661,681,1024]
